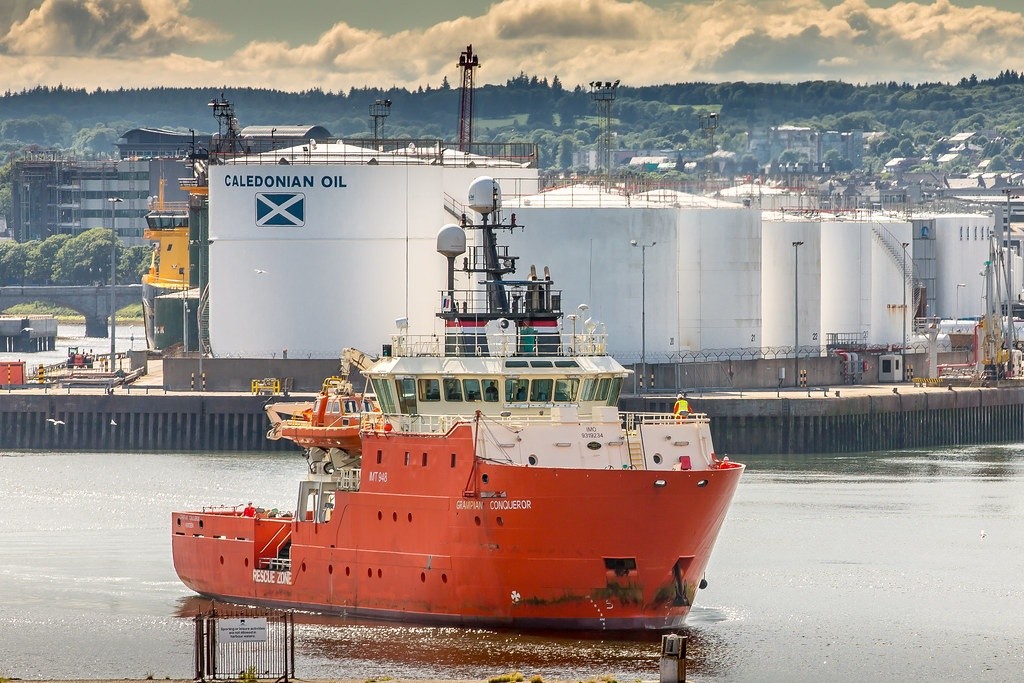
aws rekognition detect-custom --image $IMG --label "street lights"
[901,242,911,381]
[107,197,122,370]
[1001,189,1012,376]
[792,241,804,385]
[956,284,967,324]
[630,239,658,393]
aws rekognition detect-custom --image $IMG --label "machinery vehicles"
[455,44,481,153]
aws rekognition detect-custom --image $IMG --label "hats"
[677,394,683,400]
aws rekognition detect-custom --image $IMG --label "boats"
[263,176,637,459]
[171,412,746,632]
[141,129,209,346]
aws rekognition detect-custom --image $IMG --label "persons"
[486,382,497,400]
[515,386,526,401]
[674,394,694,423]
